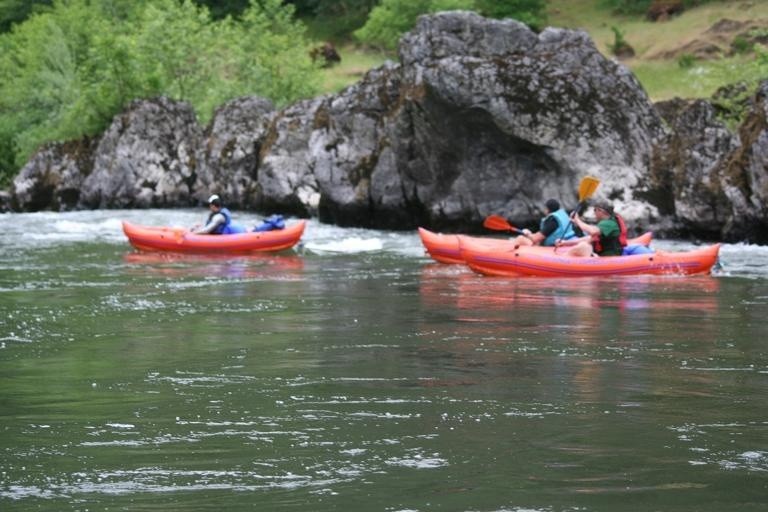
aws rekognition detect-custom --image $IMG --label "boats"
[120,218,308,255]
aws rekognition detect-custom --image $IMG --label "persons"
[553,199,624,259]
[192,193,232,236]
[515,198,576,247]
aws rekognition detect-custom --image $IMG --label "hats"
[594,197,614,214]
[544,199,559,210]
[207,194,219,203]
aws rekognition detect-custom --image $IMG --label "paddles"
[483,214,524,235]
[555,176,600,250]
